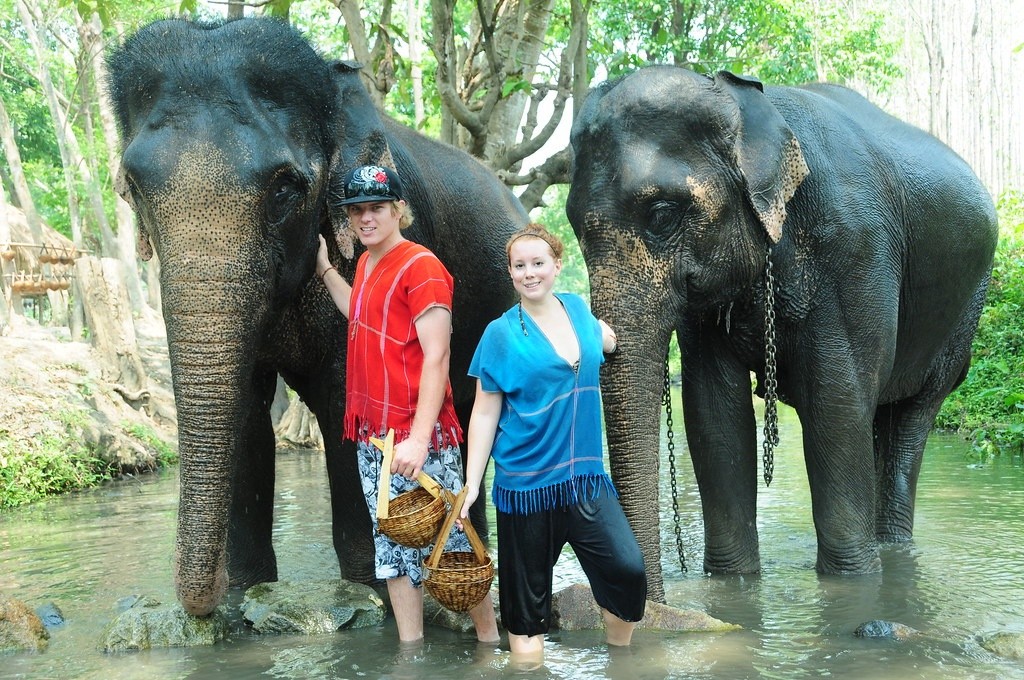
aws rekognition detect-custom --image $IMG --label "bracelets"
[322,267,337,280]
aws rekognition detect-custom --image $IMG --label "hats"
[334,165,402,208]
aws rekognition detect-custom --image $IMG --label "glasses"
[343,181,401,202]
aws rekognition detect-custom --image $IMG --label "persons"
[460,225,647,653]
[317,164,500,643]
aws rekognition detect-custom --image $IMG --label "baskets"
[369,428,447,548]
[421,485,495,615]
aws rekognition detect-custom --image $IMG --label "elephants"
[101,13,534,618]
[564,61,1000,606]
